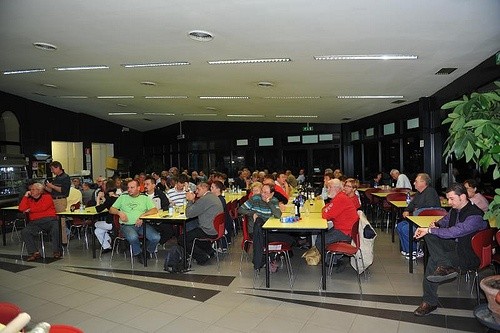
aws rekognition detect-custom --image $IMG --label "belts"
[53,197,65,199]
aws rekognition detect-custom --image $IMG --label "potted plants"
[441,82,500,317]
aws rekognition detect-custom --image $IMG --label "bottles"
[356,174,360,183]
[406,192,411,204]
[222,185,225,198]
[294,196,300,217]
[168,202,173,216]
[280,216,302,223]
[298,180,315,200]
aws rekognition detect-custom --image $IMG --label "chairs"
[9,189,500,307]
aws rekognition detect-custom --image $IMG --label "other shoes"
[401,251,406,256]
[53,253,60,258]
[209,251,216,258]
[414,303,437,316]
[427,266,459,282]
[200,255,211,265]
[406,249,424,260]
[27,254,41,261]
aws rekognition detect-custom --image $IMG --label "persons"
[413,184,488,317]
[42,161,71,247]
[440,166,477,190]
[19,169,488,274]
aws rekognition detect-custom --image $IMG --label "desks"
[1,188,445,290]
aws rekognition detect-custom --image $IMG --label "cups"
[442,198,447,204]
[179,207,184,214]
